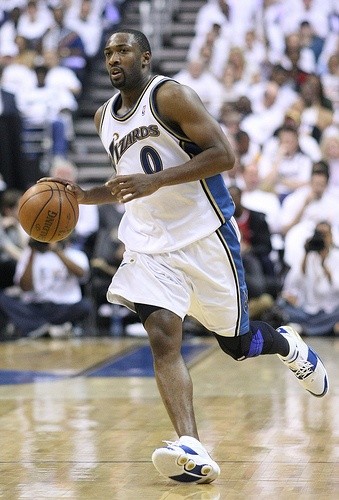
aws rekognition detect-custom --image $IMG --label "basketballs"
[16,181,79,243]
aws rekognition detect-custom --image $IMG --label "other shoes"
[49,322,83,336]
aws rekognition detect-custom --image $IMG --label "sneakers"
[275,325,329,398]
[151,435,221,484]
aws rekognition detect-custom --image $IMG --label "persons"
[0,0,339,339]
[37,29,328,486]
